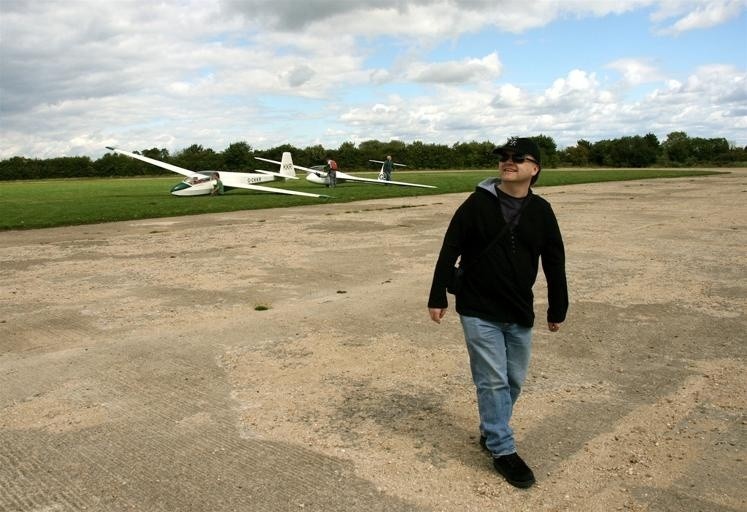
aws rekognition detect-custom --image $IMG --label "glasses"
[500,153,537,163]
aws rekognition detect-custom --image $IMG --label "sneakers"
[479,435,490,451]
[494,452,534,488]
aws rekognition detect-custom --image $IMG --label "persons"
[383,155,395,180]
[323,158,336,188]
[211,171,225,195]
[427,138,570,490]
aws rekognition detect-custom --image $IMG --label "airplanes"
[105,146,336,199]
[253,156,437,189]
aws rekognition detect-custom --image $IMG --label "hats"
[493,138,540,162]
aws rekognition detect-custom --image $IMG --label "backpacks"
[330,160,337,169]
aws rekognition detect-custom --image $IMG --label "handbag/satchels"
[447,266,461,294]
